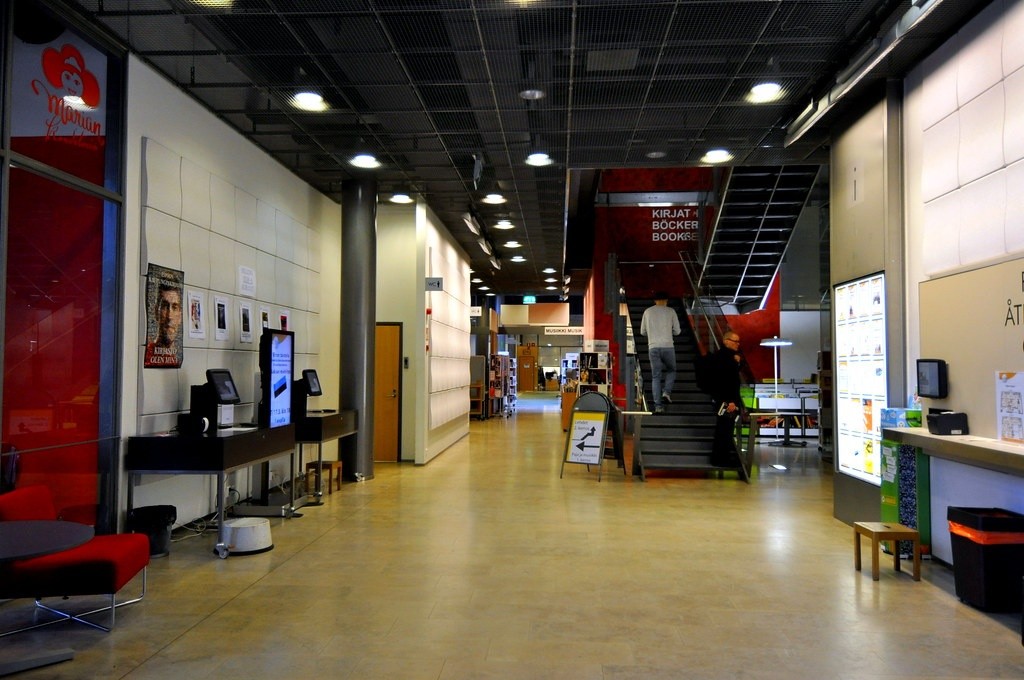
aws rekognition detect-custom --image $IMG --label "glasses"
[726,338,740,344]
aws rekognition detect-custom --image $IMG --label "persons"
[640,291,681,413]
[707,331,741,467]
[144,271,182,365]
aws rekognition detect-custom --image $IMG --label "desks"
[0,521,95,680]
[883,428,1024,476]
[261,408,359,501]
[124,427,297,557]
[768,411,812,447]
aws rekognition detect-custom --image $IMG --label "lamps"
[760,336,792,438]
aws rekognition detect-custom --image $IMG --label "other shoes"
[711,453,717,466]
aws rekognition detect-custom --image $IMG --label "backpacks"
[696,351,717,393]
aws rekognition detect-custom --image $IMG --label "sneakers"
[662,393,670,404]
[656,406,664,412]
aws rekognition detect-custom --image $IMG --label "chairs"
[0,483,151,637]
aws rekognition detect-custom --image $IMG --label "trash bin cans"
[130,505,177,559]
[947,506,1024,613]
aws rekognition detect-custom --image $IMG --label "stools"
[306,460,343,494]
[854,521,921,582]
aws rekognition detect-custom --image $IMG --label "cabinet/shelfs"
[469,355,517,420]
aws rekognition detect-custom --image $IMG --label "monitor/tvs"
[917,358,948,399]
[258,327,295,428]
[302,369,322,395]
[206,368,240,404]
